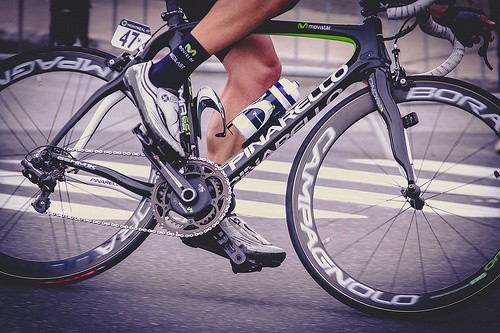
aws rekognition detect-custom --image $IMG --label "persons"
[123,0,495,267]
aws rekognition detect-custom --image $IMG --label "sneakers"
[125,60,187,155]
[183,209,285,263]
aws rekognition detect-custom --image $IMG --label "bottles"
[230,78,302,142]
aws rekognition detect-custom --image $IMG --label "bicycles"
[1,0,500,311]
